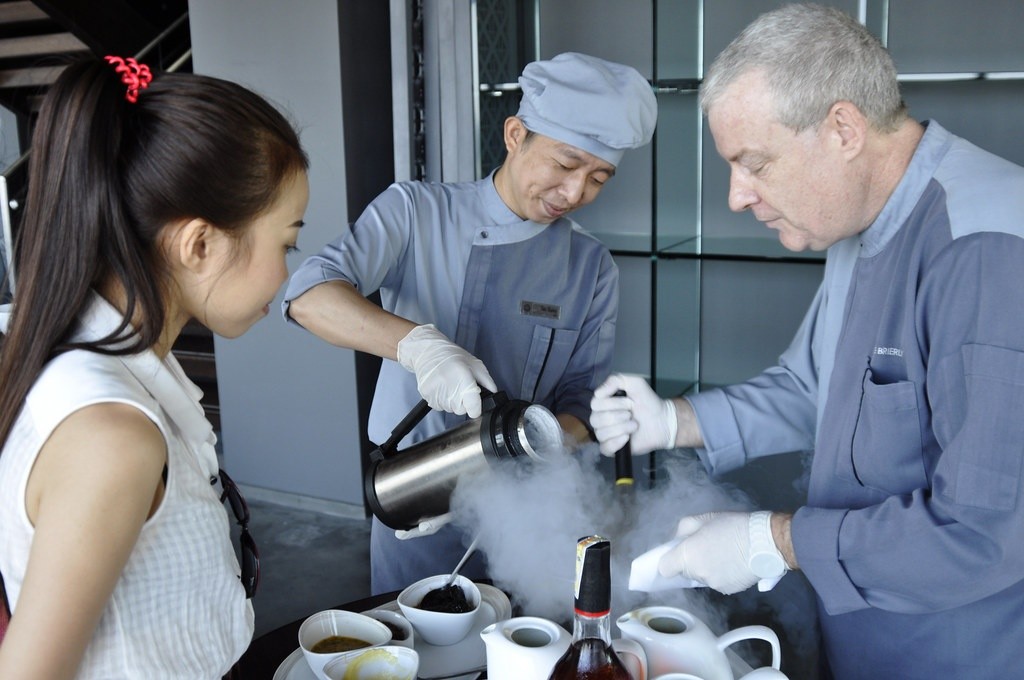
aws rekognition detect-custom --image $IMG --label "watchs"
[749,509,791,579]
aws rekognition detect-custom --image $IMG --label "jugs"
[616,606,781,680]
[480,616,647,680]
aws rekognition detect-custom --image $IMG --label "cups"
[298,610,392,680]
[360,610,414,649]
[397,574,481,645]
[323,646,420,680]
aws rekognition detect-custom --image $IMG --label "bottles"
[548,534,633,680]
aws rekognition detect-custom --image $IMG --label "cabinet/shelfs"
[469,0,1024,515]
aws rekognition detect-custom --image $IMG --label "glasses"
[212,469,259,599]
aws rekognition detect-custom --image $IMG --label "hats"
[517,51,658,168]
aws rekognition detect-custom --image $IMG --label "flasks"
[363,383,564,530]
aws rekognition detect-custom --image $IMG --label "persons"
[589,0,1024,680]
[282,51,663,598]
[0,50,312,680]
